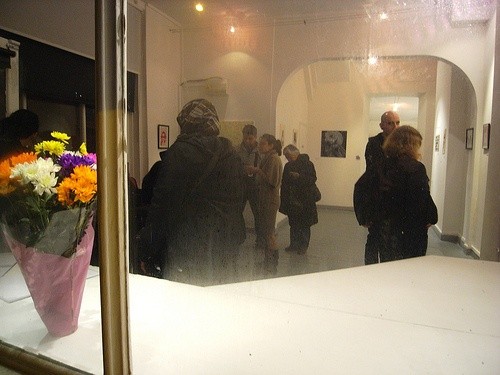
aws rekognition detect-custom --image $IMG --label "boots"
[258,248,270,269]
[269,249,279,274]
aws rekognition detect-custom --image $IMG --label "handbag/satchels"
[302,183,322,202]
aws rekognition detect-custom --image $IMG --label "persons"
[137,99,249,287]
[364,110,402,266]
[278,143,318,256]
[0,109,41,161]
[370,126,437,262]
[245,134,283,253]
[237,125,267,250]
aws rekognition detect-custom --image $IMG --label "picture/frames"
[466,128,474,149]
[158,124,169,149]
[482,123,490,149]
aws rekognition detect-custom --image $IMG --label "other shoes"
[298,247,306,255]
[285,245,297,251]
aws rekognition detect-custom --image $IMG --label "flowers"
[0,132,96,339]
